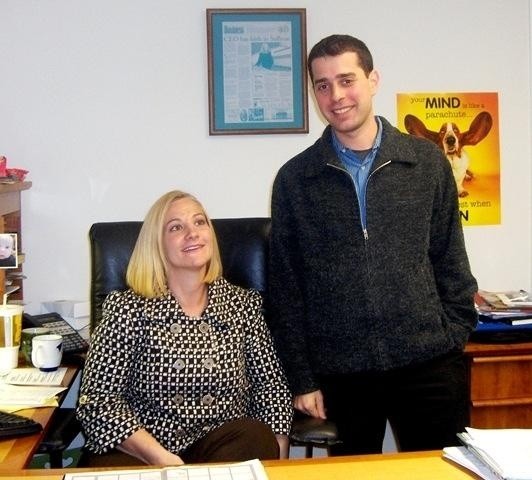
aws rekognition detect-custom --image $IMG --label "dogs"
[404,111,494,198]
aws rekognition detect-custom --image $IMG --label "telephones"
[19,312,90,354]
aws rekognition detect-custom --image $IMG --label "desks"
[0,358,476,480]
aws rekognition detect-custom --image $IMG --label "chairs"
[87,219,334,450]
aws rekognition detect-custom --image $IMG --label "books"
[442,426,532,480]
[59,457,269,480]
[0,365,70,415]
[472,287,532,332]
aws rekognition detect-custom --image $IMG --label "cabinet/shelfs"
[0,178,34,306]
[419,282,532,427]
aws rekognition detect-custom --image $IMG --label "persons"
[74,190,297,467]
[0,234,16,267]
[264,34,478,456]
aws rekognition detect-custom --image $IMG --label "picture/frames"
[206,7,309,135]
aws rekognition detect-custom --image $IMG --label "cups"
[22,327,49,364]
[31,335,63,372]
